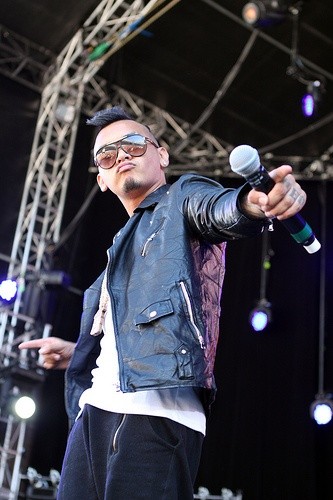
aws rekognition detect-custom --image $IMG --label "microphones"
[229,144,321,254]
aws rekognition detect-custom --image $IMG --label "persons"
[18,108,306,500]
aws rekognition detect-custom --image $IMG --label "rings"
[281,178,306,204]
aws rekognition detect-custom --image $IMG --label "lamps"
[309,181,333,427]
[249,223,271,332]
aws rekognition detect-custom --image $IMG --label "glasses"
[94,132,159,170]
